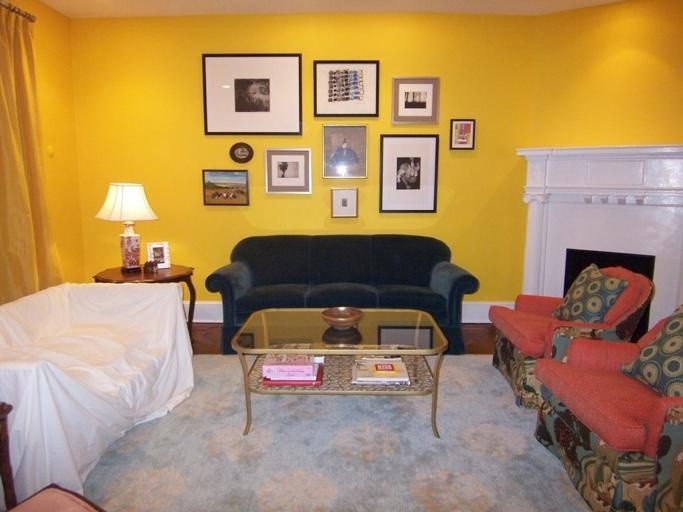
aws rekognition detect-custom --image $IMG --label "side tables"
[93,264,195,346]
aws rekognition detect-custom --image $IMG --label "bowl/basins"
[322,326,361,345]
[321,306,363,329]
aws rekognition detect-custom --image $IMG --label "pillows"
[548,263,628,323]
[620,304,683,397]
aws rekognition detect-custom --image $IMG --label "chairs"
[533,304,683,512]
[0,402,108,512]
[489,263,655,410]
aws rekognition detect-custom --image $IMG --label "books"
[351,364,410,386]
[260,354,323,387]
[355,356,408,382]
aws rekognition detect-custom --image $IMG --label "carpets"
[84,354,593,512]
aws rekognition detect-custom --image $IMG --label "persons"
[395,158,420,189]
[209,191,237,199]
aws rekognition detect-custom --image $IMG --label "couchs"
[0,282,193,512]
[205,235,480,354]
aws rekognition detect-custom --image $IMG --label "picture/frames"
[203,169,249,206]
[379,134,440,215]
[203,54,303,136]
[330,187,359,219]
[263,148,313,195]
[449,119,476,150]
[147,242,170,268]
[322,124,368,178]
[391,76,441,125]
[313,58,380,118]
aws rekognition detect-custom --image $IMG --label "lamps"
[95,183,159,273]
